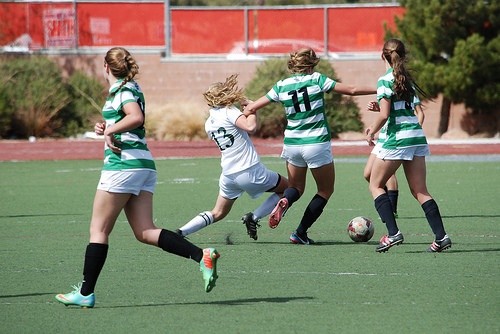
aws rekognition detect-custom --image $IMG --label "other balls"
[29,136,36,144]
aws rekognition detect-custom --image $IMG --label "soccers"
[348,216,375,243]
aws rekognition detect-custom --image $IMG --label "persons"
[243,48,378,245]
[54,46,220,309]
[364,39,452,253]
[172,74,289,240]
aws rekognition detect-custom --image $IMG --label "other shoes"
[393,214,399,219]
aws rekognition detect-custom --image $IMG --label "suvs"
[230,39,343,54]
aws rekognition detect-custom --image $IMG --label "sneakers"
[290,233,314,244]
[55,282,95,308]
[427,235,451,253]
[269,197,289,229]
[175,229,190,240]
[375,230,404,253]
[241,212,261,241]
[199,248,220,292]
